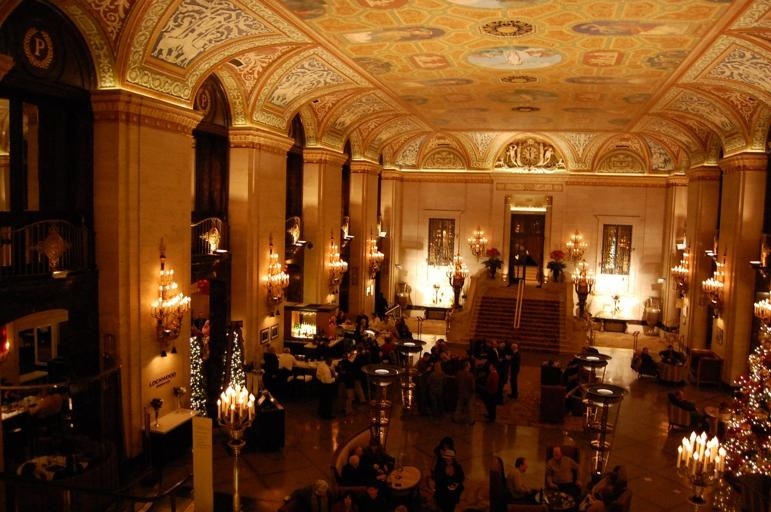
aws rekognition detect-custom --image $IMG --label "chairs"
[668,391,694,431]
[631,348,658,382]
[545,443,580,488]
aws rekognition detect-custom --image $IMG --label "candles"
[676,431,726,473]
[217,384,256,424]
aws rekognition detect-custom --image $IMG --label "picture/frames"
[260,327,269,344]
[270,323,279,340]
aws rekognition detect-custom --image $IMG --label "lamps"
[671,251,692,298]
[148,251,195,349]
[468,228,490,260]
[327,241,350,294]
[753,297,771,321]
[702,271,727,316]
[572,257,597,319]
[368,231,387,295]
[567,231,590,269]
[261,242,291,318]
[443,247,468,308]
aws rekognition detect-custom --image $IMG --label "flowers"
[546,249,568,270]
[481,248,504,268]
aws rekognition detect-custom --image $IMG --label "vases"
[173,386,187,412]
[151,398,164,426]
[489,265,497,279]
[554,268,560,282]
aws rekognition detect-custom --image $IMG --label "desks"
[140,407,203,467]
[688,347,724,387]
[385,463,424,500]
[263,319,404,386]
[16,455,88,512]
[1,396,27,421]
[217,387,286,454]
[658,361,687,382]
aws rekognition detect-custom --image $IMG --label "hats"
[441,449,458,460]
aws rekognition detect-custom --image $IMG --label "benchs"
[489,455,549,512]
[586,471,631,512]
[330,424,374,484]
